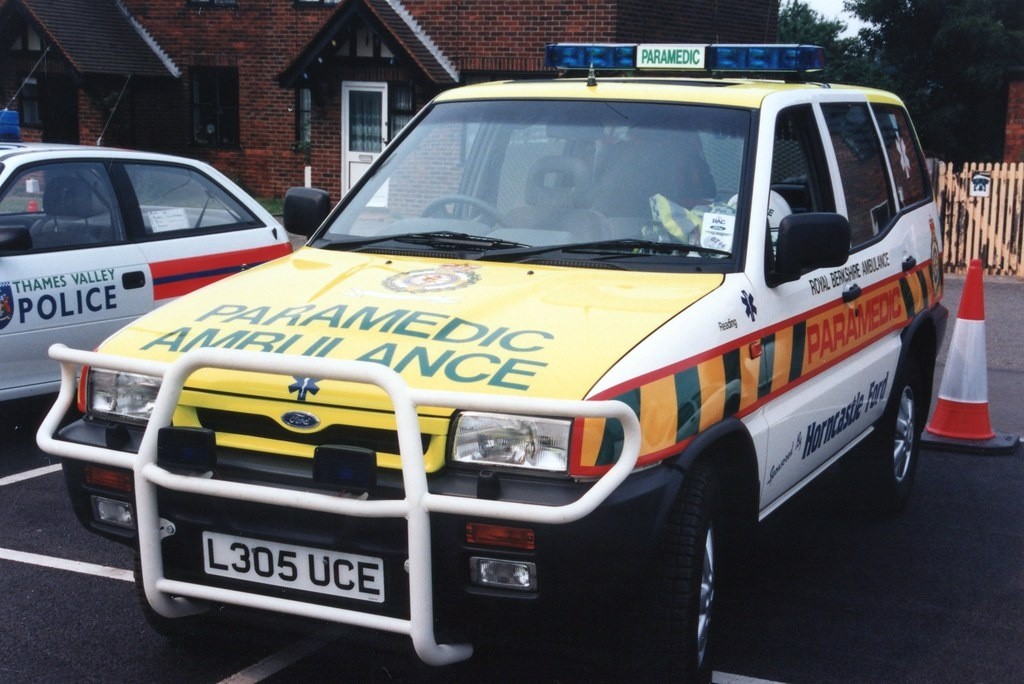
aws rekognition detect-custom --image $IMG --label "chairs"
[29,176,115,250]
[772,184,812,214]
[505,155,610,240]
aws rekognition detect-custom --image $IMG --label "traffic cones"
[923,258,1023,453]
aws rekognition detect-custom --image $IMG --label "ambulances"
[33,39,955,683]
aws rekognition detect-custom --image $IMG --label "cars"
[0,108,297,405]
